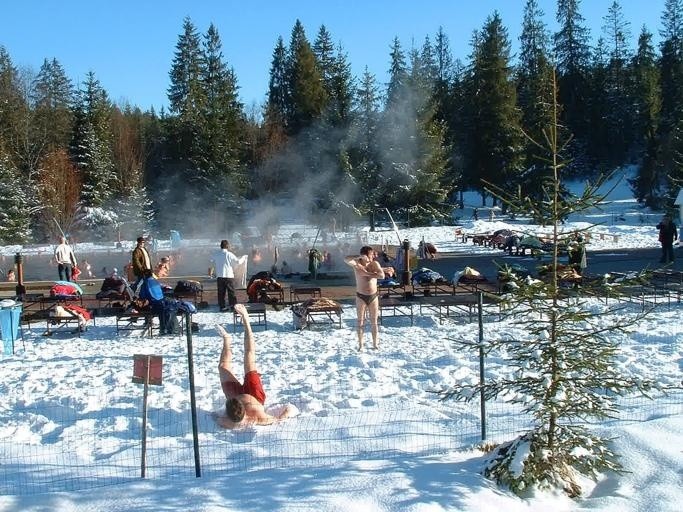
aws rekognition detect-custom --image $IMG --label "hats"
[137,237,145,242]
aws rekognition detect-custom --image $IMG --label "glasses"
[139,241,145,244]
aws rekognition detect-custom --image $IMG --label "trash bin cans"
[170,230,180,254]
[0,301,25,356]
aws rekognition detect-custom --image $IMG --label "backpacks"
[412,266,489,285]
[95,275,203,335]
[50,281,83,298]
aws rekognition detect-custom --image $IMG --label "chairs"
[22,266,544,340]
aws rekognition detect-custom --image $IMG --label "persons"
[96,229,182,334]
[455,229,465,241]
[212,240,248,310]
[343,245,385,352]
[569,229,587,288]
[208,216,436,278]
[6,237,92,281]
[488,209,497,222]
[211,304,289,428]
[473,208,479,222]
[656,214,677,262]
